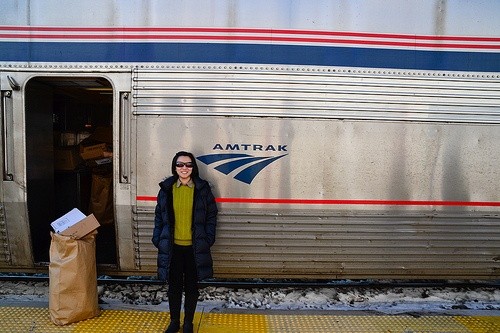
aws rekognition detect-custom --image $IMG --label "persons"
[152,150,222,333]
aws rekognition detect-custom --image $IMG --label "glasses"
[176,162,193,167]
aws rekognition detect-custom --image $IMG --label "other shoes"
[165,322,180,333]
[183,323,193,333]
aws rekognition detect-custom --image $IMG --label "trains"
[0,1,500,284]
